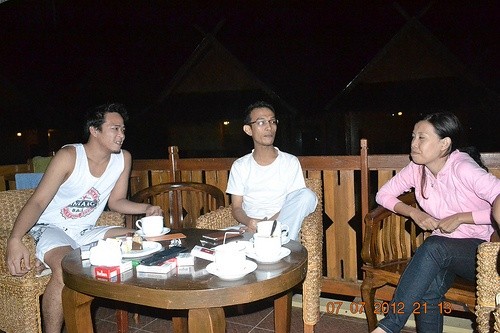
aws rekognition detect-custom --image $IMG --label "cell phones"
[141,247,188,266]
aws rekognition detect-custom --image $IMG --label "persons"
[371,112,500,333]
[7,103,164,333]
[226,102,318,241]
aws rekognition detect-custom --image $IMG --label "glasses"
[248,120,279,126]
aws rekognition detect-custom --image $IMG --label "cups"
[254,233,281,258]
[136,216,163,235]
[215,244,246,272]
[257,221,289,241]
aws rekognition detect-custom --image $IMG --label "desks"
[61,227,309,333]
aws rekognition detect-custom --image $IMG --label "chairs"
[361,160,500,333]
[197,176,323,333]
[129,181,225,229]
[0,189,125,333]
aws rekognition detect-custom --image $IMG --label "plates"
[206,260,257,280]
[246,247,291,263]
[136,227,171,237]
[252,236,290,246]
[122,241,162,259]
